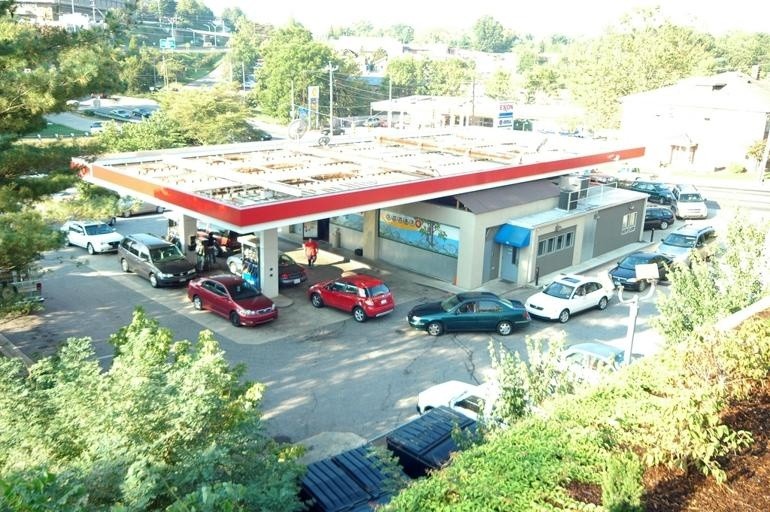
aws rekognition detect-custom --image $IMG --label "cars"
[306,274,394,322]
[525,275,613,323]
[187,273,278,328]
[60,220,123,254]
[407,292,532,336]
[644,207,674,230]
[227,247,307,288]
[628,181,671,205]
[608,252,673,292]
[109,108,150,118]
[117,198,165,218]
[321,127,344,135]
[552,341,624,387]
[254,128,271,141]
[363,117,383,127]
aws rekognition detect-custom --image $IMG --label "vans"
[117,232,197,288]
[655,224,716,268]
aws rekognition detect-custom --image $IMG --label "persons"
[304,237,319,267]
[466,304,473,313]
[208,232,218,263]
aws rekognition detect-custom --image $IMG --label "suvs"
[196,221,255,255]
[670,184,708,220]
[417,380,506,427]
